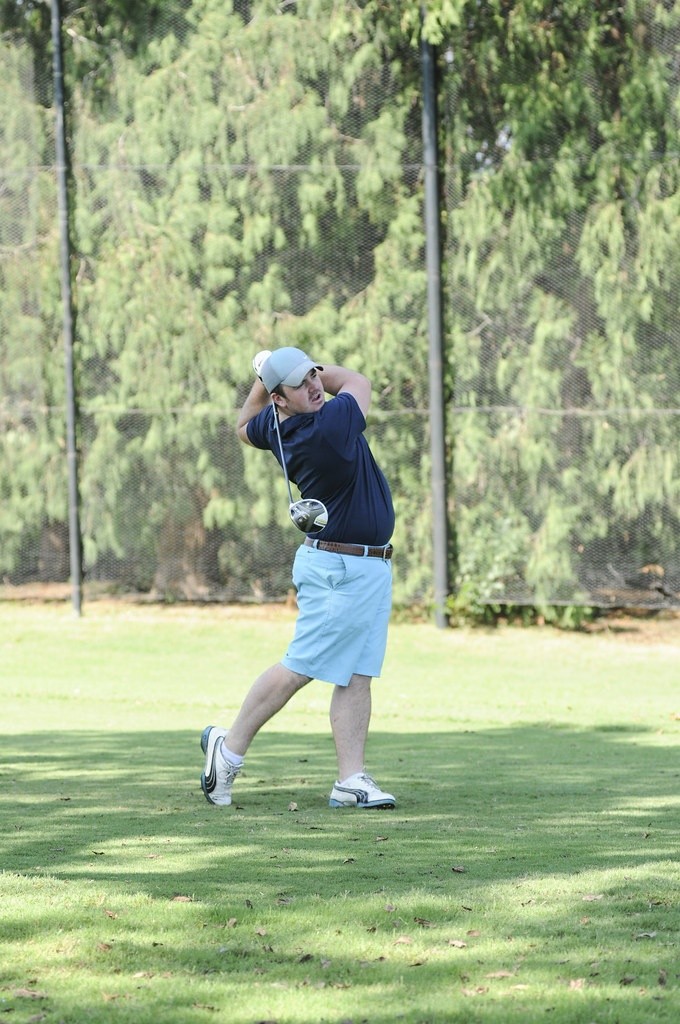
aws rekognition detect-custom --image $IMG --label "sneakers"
[199,725,245,808]
[329,766,397,809]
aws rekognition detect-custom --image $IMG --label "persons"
[201,346,396,809]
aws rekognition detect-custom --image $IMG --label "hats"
[259,346,324,395]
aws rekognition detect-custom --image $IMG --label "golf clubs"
[269,400,329,536]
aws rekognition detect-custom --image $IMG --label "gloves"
[251,350,272,379]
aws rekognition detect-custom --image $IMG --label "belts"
[302,535,394,560]
